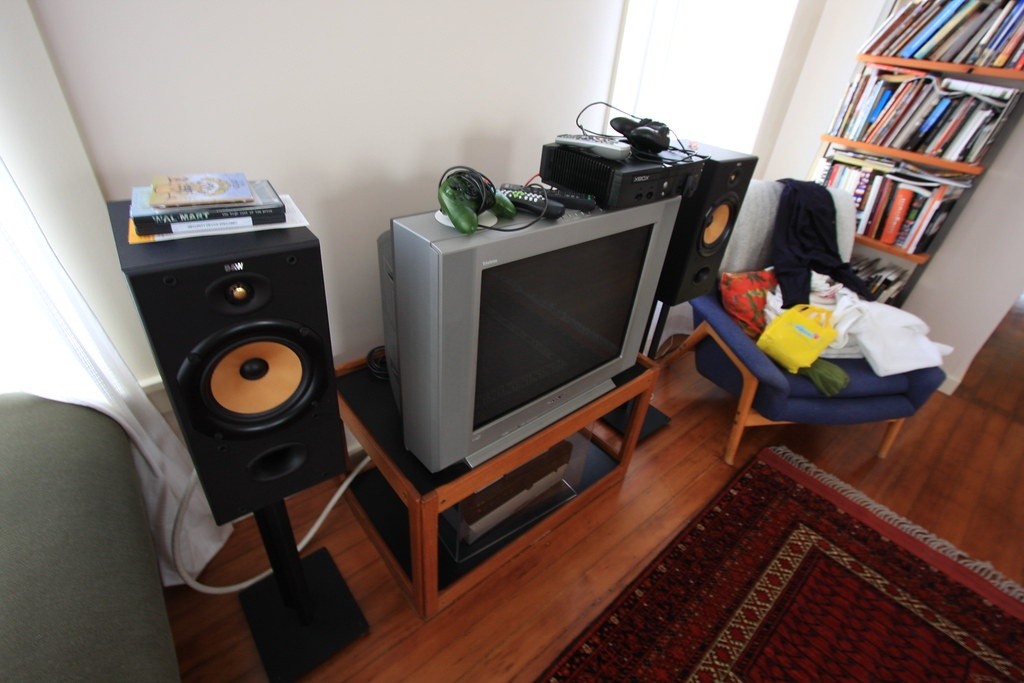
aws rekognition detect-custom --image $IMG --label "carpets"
[538,444,1024,682]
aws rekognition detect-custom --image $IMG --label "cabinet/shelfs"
[335,354,663,622]
[820,53,1024,309]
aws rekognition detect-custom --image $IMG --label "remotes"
[555,134,632,161]
[497,182,596,219]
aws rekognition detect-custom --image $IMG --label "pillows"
[718,268,784,338]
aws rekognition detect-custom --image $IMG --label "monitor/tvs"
[378,186,683,484]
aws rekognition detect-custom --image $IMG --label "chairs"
[660,177,955,466]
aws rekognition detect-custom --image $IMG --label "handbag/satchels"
[755,304,838,374]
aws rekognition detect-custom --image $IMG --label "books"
[805,0,1024,305]
[128,173,310,244]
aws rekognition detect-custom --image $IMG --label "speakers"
[107,197,350,524]
[655,142,758,307]
